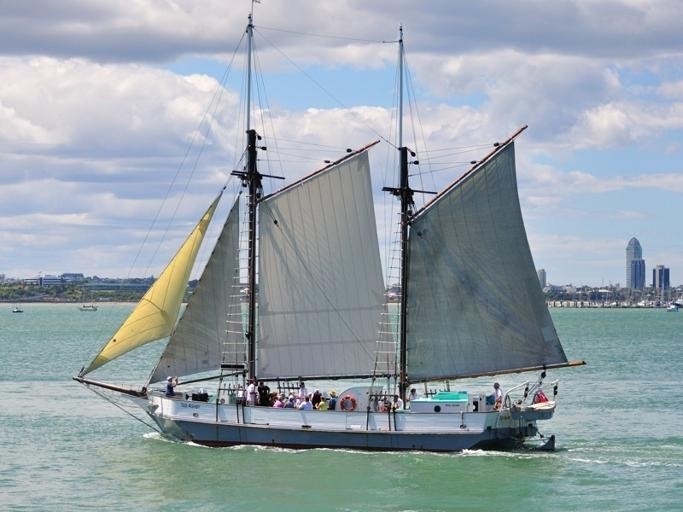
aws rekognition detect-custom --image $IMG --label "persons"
[236,380,418,411]
[166,375,179,395]
[493,383,503,409]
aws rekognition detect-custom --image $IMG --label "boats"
[667,305,678,311]
[79,306,96,311]
[12,310,23,313]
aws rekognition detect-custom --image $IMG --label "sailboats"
[71,1,587,453]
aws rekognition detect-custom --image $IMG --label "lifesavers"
[339,396,356,411]
